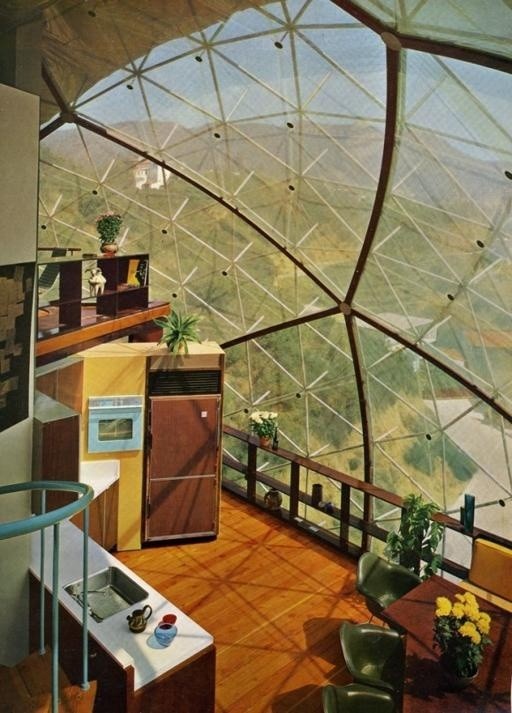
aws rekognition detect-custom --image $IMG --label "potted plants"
[154,309,201,360]
[95,211,122,254]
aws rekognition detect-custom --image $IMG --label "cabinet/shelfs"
[36,253,148,341]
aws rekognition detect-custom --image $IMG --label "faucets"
[78,590,105,601]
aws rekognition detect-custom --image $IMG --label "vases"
[261,435,270,446]
[451,668,479,689]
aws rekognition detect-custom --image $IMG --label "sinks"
[63,567,149,623]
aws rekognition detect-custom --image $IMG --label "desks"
[382,576,512,713]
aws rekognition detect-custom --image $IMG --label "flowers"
[432,590,493,678]
[250,411,278,437]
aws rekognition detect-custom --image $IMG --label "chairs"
[339,620,403,693]
[356,552,421,634]
[321,683,400,713]
[458,538,512,613]
